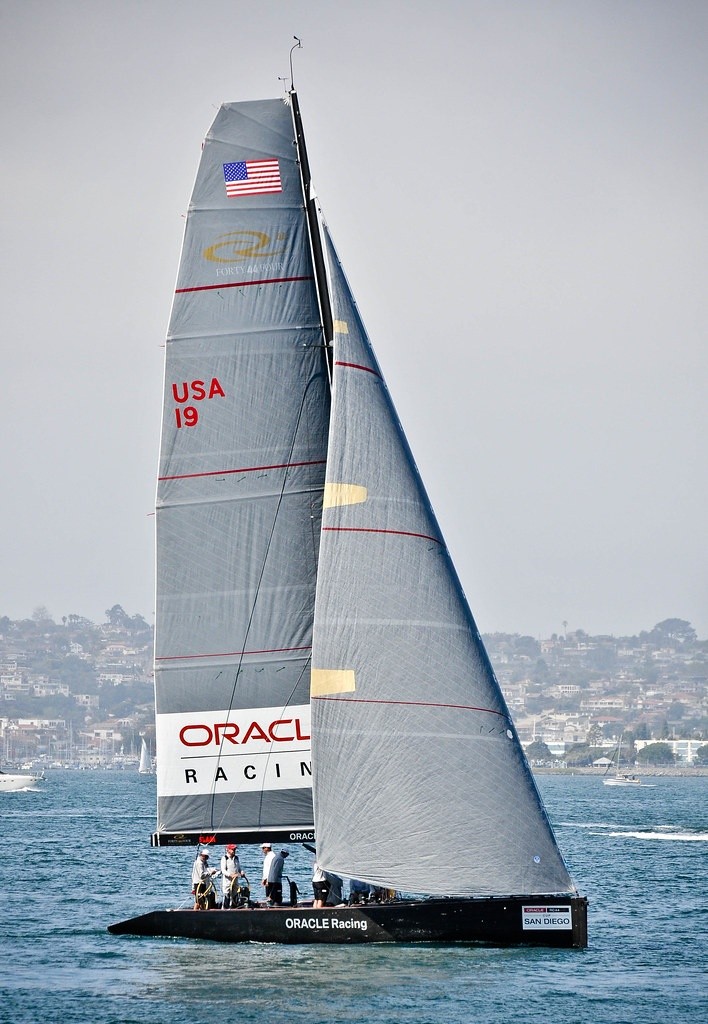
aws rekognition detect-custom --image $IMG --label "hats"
[226,844,237,850]
[201,849,210,855]
[258,843,271,848]
[281,848,289,854]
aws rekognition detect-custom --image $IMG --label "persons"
[259,843,290,909]
[312,860,397,909]
[194,849,210,911]
[220,844,245,910]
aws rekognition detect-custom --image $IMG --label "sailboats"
[0,718,136,770]
[105,34,590,951]
[599,733,641,787]
[137,738,156,773]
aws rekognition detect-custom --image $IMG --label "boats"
[0,765,46,793]
